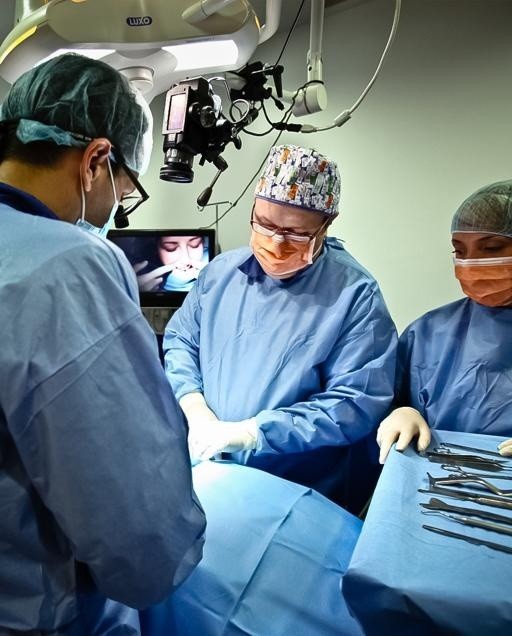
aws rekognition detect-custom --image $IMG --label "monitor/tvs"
[105,229,215,307]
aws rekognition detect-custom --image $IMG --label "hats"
[255,145,342,217]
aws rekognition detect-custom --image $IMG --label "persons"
[377,178,512,464]
[162,145,399,503]
[133,260,176,293]
[1,50,205,636]
[154,236,208,292]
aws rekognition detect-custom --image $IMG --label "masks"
[74,159,119,239]
[250,222,316,280]
[453,256,512,308]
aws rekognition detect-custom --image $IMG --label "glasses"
[107,143,150,220]
[250,203,330,245]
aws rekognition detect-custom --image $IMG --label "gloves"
[181,392,258,461]
[376,406,431,466]
